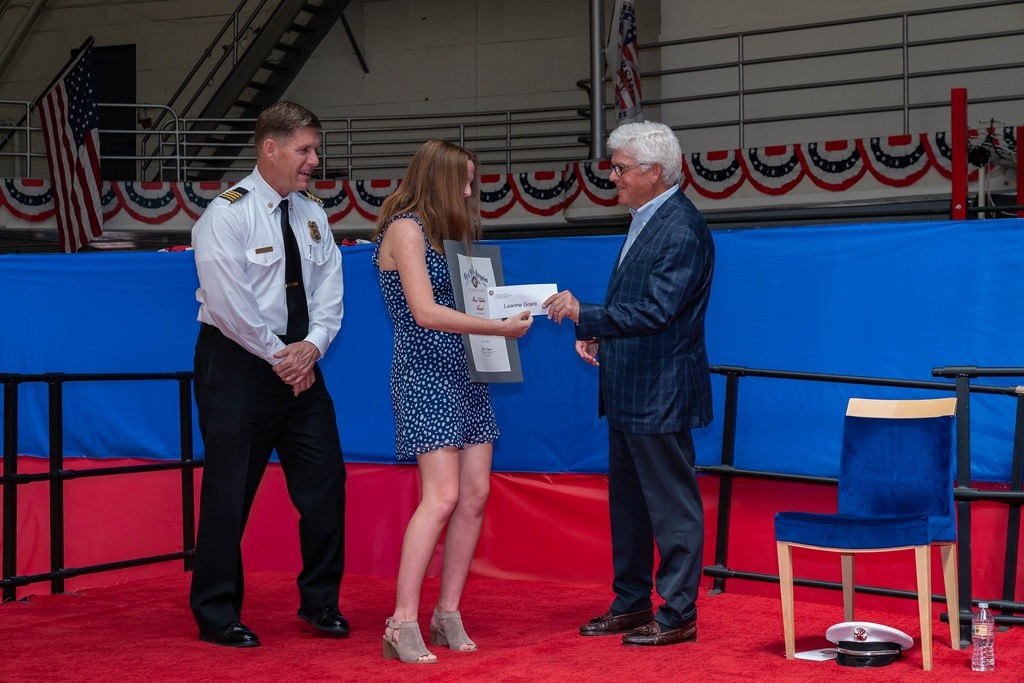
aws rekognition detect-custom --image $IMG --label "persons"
[188,101,351,646]
[542,120,716,646]
[372,138,534,664]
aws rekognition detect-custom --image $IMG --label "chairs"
[772,396,961,671]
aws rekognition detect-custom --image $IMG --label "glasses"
[610,163,645,178]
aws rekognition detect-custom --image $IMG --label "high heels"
[382,617,439,664]
[429,608,479,652]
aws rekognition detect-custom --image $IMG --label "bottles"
[972,603,995,672]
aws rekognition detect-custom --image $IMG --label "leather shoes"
[621,619,698,646]
[579,606,654,636]
[297,604,350,634]
[200,621,260,647]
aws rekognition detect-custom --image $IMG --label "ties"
[277,199,309,346]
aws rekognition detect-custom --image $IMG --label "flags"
[39,51,105,253]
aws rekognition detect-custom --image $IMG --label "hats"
[826,621,914,665]
